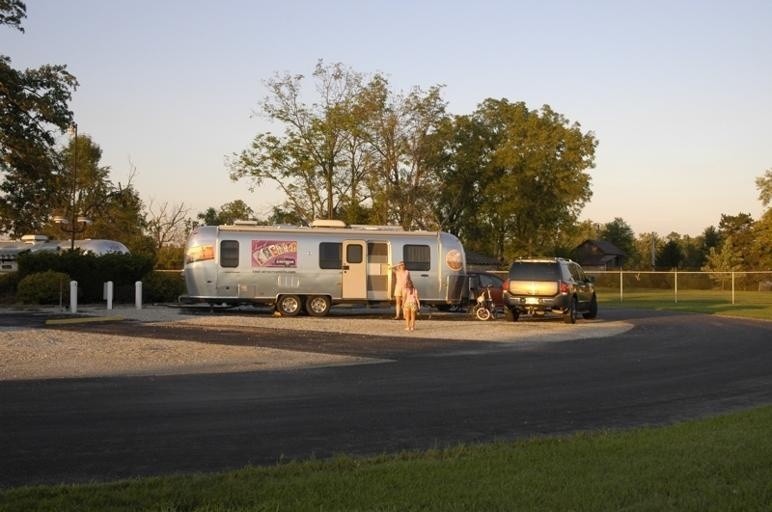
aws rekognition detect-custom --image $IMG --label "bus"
[182,221,468,317]
[1,234,130,275]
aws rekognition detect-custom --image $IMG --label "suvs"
[502,257,597,323]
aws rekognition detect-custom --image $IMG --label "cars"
[438,270,507,312]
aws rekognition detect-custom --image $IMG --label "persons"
[401,280,421,331]
[388,262,413,321]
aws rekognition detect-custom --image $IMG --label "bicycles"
[476,284,497,320]
